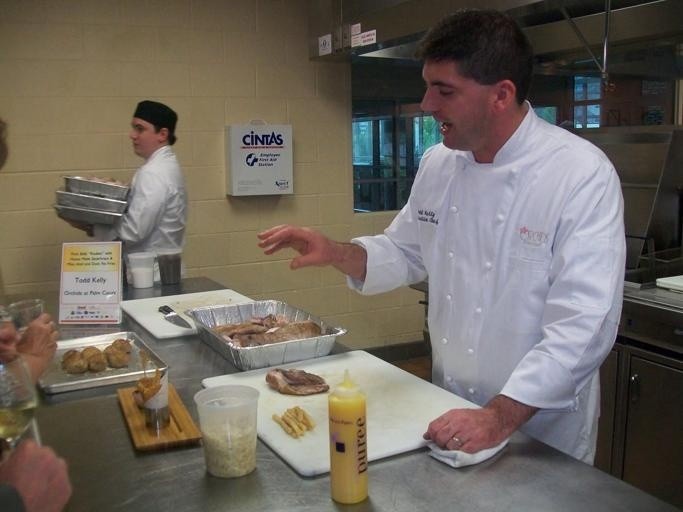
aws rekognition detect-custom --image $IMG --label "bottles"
[327,365,370,505]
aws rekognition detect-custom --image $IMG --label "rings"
[449,433,465,447]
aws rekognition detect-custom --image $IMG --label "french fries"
[273,406,316,438]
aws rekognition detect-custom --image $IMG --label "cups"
[4,297,48,334]
[193,383,259,481]
[123,245,184,287]
[144,408,171,428]
[0,350,41,452]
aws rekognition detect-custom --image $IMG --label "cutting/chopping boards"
[119,288,261,343]
[201,348,504,478]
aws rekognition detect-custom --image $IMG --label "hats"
[133,99,177,135]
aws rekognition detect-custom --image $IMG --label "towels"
[424,436,511,470]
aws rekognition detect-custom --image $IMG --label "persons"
[0,118,58,414]
[253,9,630,469]
[0,435,71,512]
[54,99,188,291]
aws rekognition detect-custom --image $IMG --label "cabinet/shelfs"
[590,339,682,508]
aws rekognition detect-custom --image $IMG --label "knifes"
[156,305,190,332]
